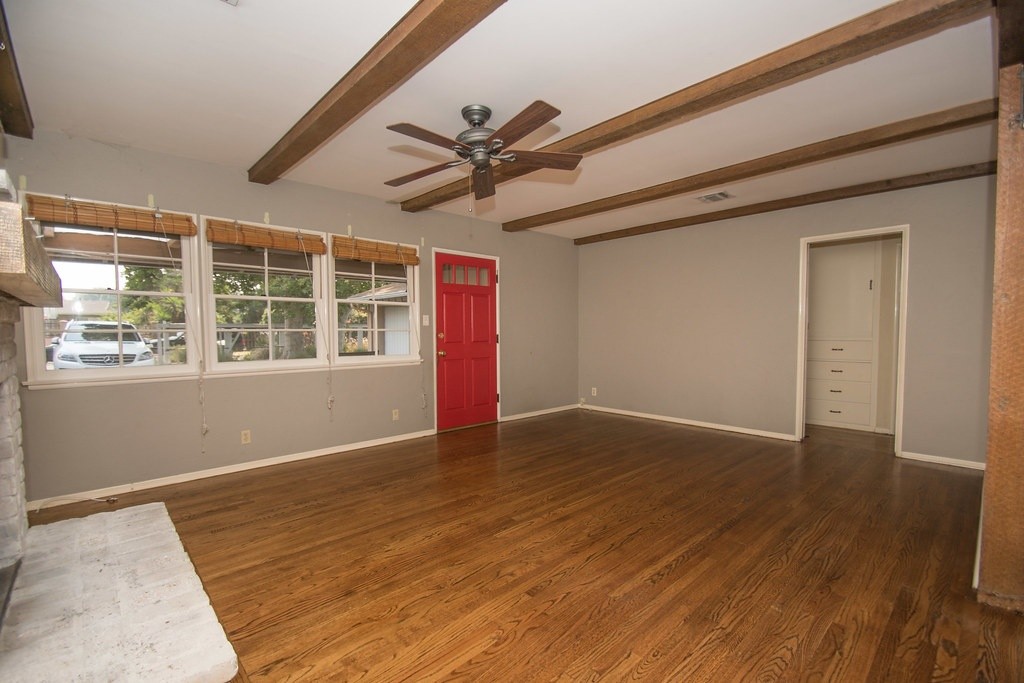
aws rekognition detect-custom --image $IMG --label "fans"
[385,101,585,201]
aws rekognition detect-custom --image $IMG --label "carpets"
[0,502,239,683]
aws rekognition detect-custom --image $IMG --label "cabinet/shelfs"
[805,240,882,431]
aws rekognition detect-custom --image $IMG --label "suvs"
[143,328,246,351]
[52,319,157,370]
